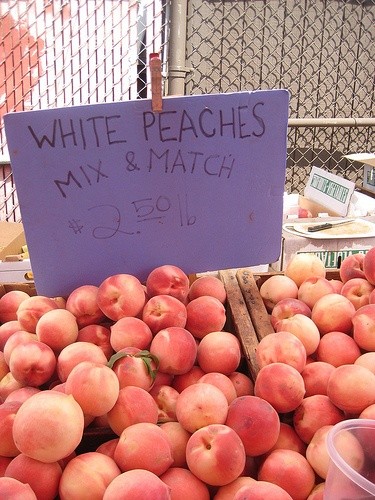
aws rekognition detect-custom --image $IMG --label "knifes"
[308,218,354,232]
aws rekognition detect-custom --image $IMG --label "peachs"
[0,246,375,500]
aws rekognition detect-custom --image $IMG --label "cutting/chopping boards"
[282,219,375,239]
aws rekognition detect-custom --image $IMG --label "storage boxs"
[339,237,375,268]
[270,224,338,272]
[341,153,375,196]
[0,267,345,387]
[0,220,31,263]
[297,166,356,218]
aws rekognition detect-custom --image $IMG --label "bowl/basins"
[322,418,375,500]
